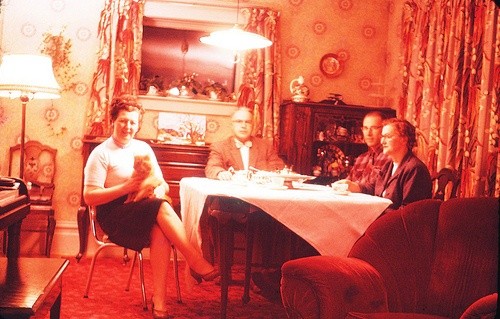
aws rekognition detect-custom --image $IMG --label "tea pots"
[290,76,311,102]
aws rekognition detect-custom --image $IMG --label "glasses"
[361,126,383,131]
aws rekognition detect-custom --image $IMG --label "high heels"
[150,295,169,319]
[190,269,221,283]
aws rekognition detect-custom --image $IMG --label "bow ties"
[235,141,252,148]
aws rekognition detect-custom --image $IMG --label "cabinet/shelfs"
[278,101,396,179]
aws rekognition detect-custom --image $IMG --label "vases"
[210,90,217,99]
[181,86,188,96]
[148,85,156,94]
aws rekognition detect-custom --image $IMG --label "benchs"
[0,256,70,319]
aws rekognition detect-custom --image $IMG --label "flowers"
[140,72,228,99]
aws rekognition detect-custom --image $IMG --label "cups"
[332,184,348,192]
[271,177,285,187]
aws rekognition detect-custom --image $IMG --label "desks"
[75,136,210,262]
[179,177,394,319]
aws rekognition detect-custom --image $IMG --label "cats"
[122,155,172,205]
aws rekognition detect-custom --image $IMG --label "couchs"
[279,196,499,319]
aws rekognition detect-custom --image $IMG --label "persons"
[334,119,432,210]
[334,111,387,196]
[83,97,220,319]
[205,107,286,284]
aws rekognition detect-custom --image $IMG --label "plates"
[270,186,288,190]
[332,190,350,195]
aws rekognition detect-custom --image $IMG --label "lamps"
[199,0,272,51]
[0,53,62,179]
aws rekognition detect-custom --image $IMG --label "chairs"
[8,140,58,258]
[83,204,182,312]
[431,167,460,201]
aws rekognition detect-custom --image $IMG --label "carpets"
[0,252,288,319]
